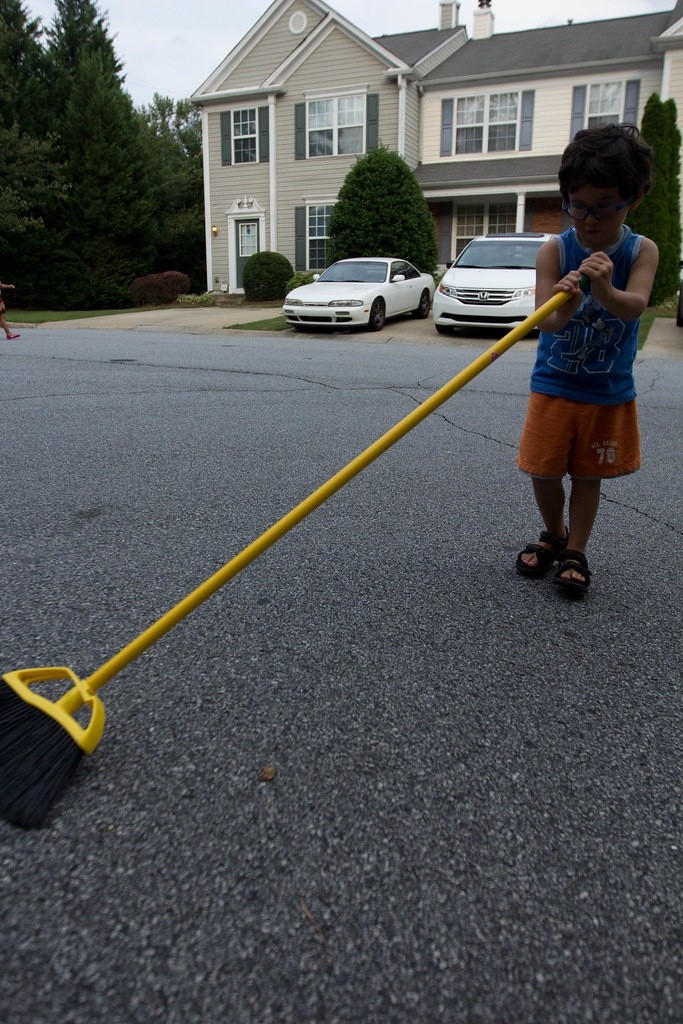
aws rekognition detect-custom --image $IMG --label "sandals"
[553,549,593,593]
[515,526,569,578]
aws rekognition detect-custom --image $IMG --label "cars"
[280,256,436,332]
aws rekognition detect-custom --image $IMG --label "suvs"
[432,232,563,340]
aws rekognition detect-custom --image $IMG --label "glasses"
[564,191,639,222]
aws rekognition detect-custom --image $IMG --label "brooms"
[0,272,602,832]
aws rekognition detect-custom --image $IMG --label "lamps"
[213,225,218,236]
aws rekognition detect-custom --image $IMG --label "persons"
[514,121,660,595]
[0,281,21,340]
